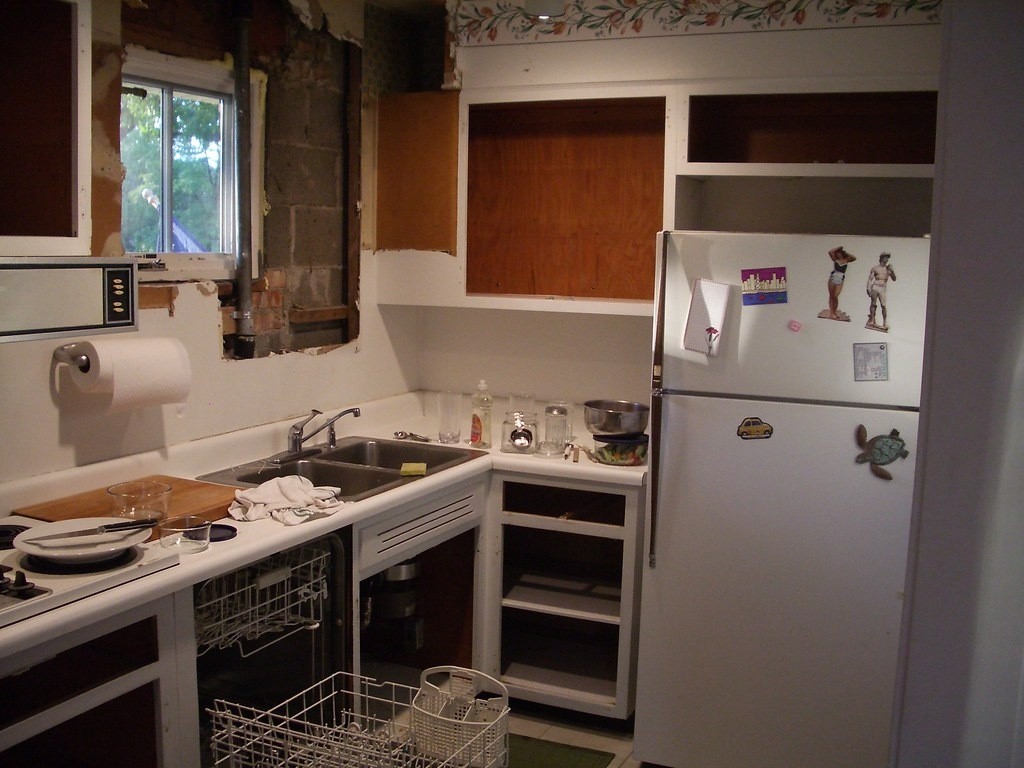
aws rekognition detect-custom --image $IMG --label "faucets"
[288,407,361,454]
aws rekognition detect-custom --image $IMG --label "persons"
[866,251,897,328]
[828,245,856,319]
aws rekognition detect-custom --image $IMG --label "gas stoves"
[0,514,179,628]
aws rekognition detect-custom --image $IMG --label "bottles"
[543,406,567,448]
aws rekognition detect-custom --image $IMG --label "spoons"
[395,431,431,442]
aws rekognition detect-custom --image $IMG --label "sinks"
[308,435,489,471]
[195,458,414,504]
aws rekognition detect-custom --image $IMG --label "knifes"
[22,518,158,542]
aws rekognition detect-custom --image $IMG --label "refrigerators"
[633,229,932,768]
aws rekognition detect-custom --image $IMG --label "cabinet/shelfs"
[471,472,655,724]
[452,82,943,314]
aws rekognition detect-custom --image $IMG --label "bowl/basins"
[585,400,650,439]
[107,481,173,522]
[593,434,650,467]
[157,516,212,554]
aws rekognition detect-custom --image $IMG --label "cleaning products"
[469,379,493,449]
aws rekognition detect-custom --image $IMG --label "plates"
[14,518,152,564]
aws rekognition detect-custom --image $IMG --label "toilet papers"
[70,338,189,415]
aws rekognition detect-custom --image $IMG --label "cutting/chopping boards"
[10,475,248,543]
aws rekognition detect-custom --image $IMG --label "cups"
[439,392,464,444]
[553,401,573,442]
[509,391,536,410]
